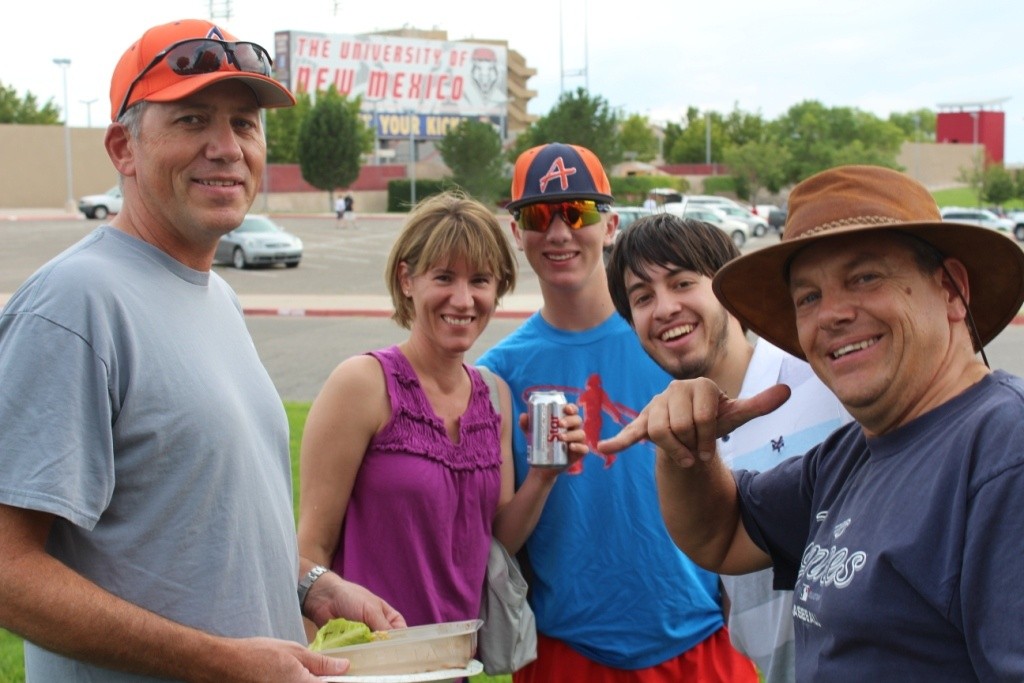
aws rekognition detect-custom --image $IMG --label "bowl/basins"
[320,618,485,675]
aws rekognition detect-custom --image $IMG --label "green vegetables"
[310,616,372,654]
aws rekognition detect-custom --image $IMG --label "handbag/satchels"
[476,536,539,677]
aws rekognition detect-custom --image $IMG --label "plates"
[316,659,485,683]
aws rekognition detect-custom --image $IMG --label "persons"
[336,195,346,219]
[472,141,763,683]
[598,165,1024,683]
[642,193,657,209]
[606,215,857,683]
[0,18,411,683]
[297,182,588,683]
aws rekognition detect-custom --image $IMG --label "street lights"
[52,57,75,211]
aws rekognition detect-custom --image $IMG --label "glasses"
[115,38,274,122]
[513,198,613,232]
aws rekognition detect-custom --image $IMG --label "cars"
[215,215,304,270]
[943,207,1024,240]
[79,186,124,221]
[611,195,786,251]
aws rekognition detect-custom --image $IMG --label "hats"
[110,19,300,122]
[710,162,1024,363]
[503,142,619,216]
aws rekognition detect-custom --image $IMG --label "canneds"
[527,391,568,470]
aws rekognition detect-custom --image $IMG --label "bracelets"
[297,565,329,607]
